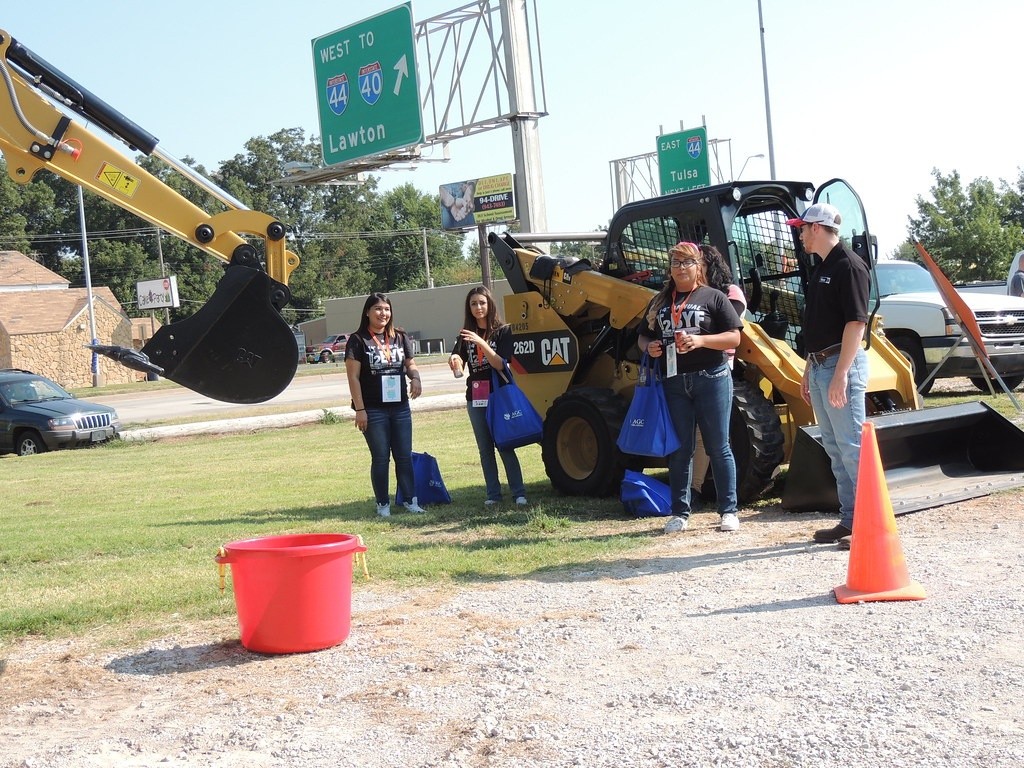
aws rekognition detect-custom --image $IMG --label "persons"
[1007,245,1024,295]
[344,293,428,517]
[448,286,527,505]
[690,245,747,504]
[440,179,478,227]
[786,203,871,549]
[637,242,739,533]
[1010,254,1024,298]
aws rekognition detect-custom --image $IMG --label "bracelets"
[449,198,456,210]
[466,182,476,191]
[355,409,366,411]
[411,377,420,382]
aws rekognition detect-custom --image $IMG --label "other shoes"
[840,535,850,549]
[813,524,850,543]
[690,488,702,506]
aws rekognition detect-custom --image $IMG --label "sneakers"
[665,516,687,533]
[721,512,739,531]
[377,502,390,516]
[484,500,497,504]
[403,497,427,513]
[516,497,527,504]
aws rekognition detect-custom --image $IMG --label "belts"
[809,344,841,363]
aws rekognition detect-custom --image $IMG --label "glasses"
[669,261,696,268]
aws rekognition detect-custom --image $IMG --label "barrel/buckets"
[214,532,368,655]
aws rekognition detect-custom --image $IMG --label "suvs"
[0,367,123,456]
[767,259,1024,391]
[306,333,351,363]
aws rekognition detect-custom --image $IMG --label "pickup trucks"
[954,249,1024,296]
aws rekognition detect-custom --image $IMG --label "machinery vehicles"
[487,181,1024,516]
[1,29,299,406]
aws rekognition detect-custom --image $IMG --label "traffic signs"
[312,0,425,168]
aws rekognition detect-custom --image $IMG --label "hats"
[785,203,841,229]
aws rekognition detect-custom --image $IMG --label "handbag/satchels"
[617,348,682,457]
[487,359,543,451]
[619,470,672,516]
[395,452,451,505]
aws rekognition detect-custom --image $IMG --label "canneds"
[452,358,464,377]
[674,330,688,354]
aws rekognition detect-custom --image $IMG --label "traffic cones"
[834,422,926,602]
